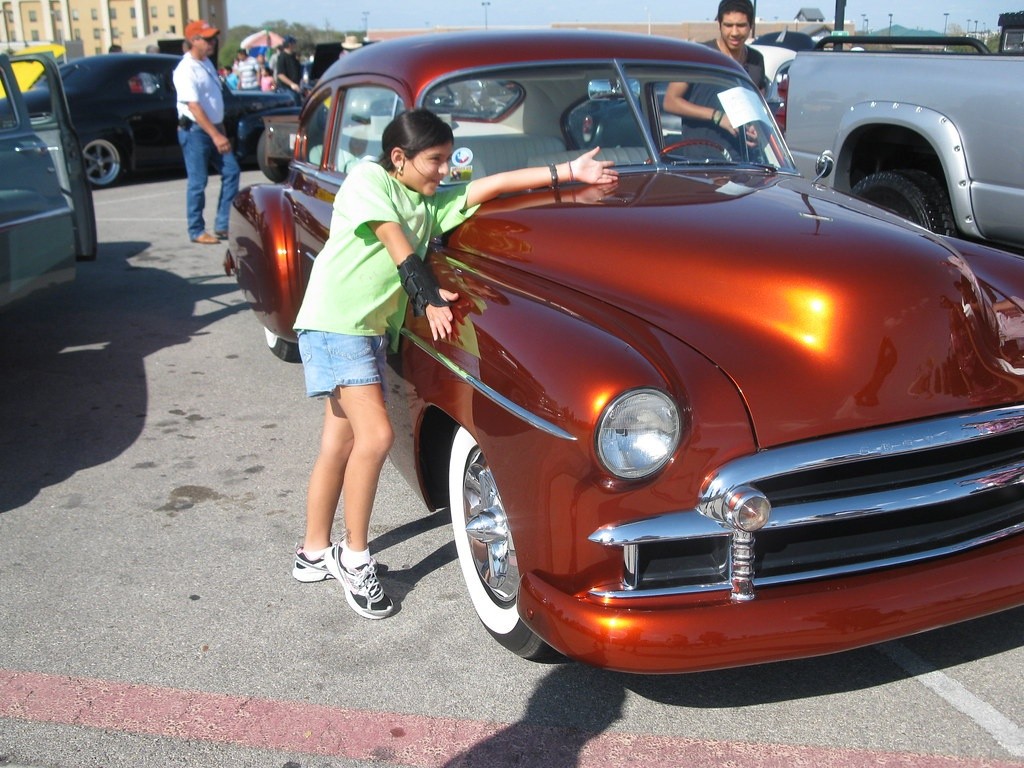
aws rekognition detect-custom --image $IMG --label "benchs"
[447,132,564,175]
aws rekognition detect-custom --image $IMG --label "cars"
[0,49,100,315]
[221,26,1024,676]
[0,52,300,189]
[563,29,810,164]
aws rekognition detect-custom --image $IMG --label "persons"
[109,45,160,93]
[340,36,363,57]
[223,37,314,92]
[293,110,619,620]
[664,0,766,161]
[173,20,240,244]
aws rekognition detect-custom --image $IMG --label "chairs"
[524,143,649,197]
[443,155,488,194]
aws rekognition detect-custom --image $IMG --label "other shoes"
[192,233,219,244]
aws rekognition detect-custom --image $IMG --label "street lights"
[864,19,869,34]
[861,13,867,32]
[887,13,893,39]
[481,2,492,30]
[974,20,979,35]
[943,12,950,36]
[967,19,971,35]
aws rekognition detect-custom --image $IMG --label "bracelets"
[712,108,724,127]
[549,163,558,188]
[569,161,574,182]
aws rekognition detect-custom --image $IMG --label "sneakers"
[325,542,395,619]
[291,543,340,582]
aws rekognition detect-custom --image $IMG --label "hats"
[341,36,362,49]
[184,22,220,42]
[282,36,295,47]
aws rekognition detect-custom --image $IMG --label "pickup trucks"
[777,23,1024,257]
